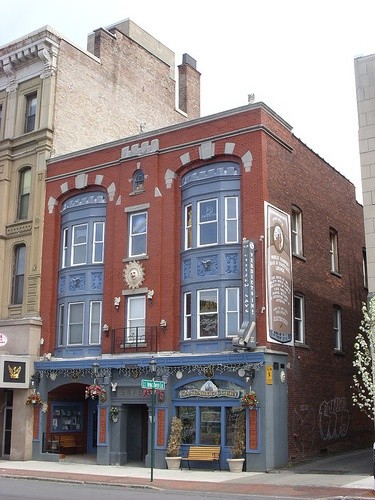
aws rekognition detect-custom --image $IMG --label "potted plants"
[164,417,183,469]
[226,414,246,472]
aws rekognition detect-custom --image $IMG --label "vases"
[92,390,97,395]
[112,416,118,423]
[249,406,253,410]
[32,398,37,404]
[150,390,156,395]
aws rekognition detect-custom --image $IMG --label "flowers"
[143,389,163,403]
[85,383,107,401]
[109,407,119,423]
[239,391,259,411]
[24,391,49,415]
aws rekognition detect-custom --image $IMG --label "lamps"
[92,363,99,376]
[43,352,52,362]
[149,356,158,372]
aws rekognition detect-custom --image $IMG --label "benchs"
[53,435,83,456]
[182,446,223,471]
